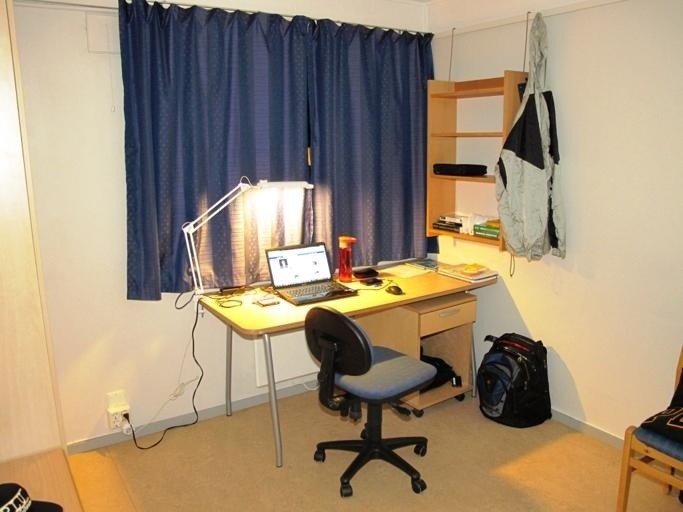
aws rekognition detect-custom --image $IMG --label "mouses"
[386,286,403,296]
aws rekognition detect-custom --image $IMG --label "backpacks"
[477,332,551,428]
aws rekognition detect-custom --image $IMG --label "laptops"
[264,241,358,306]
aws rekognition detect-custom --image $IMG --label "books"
[432,212,500,240]
[405,259,498,284]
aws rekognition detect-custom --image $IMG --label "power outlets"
[108,403,130,430]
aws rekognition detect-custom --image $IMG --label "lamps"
[181,175,315,294]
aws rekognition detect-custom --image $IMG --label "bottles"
[338,236,357,283]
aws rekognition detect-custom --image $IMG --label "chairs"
[615,349,683,511]
[302,304,438,496]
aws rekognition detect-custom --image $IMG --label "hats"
[0,484,62,512]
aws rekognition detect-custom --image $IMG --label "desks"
[198,259,501,468]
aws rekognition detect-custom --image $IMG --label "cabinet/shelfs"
[426,69,530,252]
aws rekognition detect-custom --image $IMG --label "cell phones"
[258,298,280,306]
